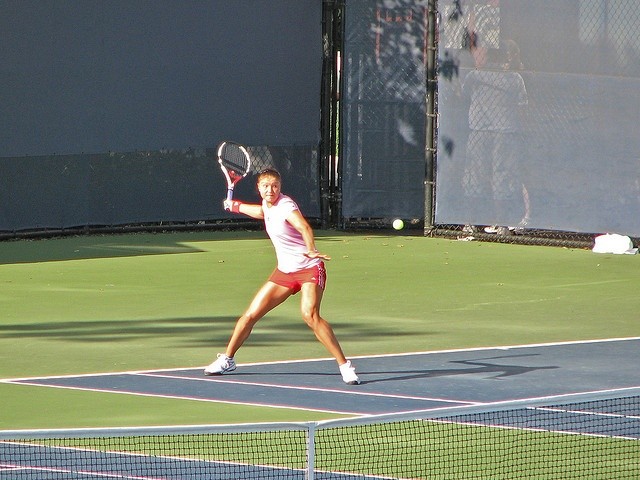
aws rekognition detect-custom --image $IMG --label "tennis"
[393,219,404,230]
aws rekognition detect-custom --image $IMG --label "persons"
[519,182,533,227]
[204,168,361,385]
[453,48,526,236]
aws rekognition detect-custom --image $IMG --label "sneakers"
[203,353,237,375]
[338,361,361,385]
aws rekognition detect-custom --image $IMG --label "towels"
[592,233,639,256]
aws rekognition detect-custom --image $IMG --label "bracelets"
[232,201,242,214]
